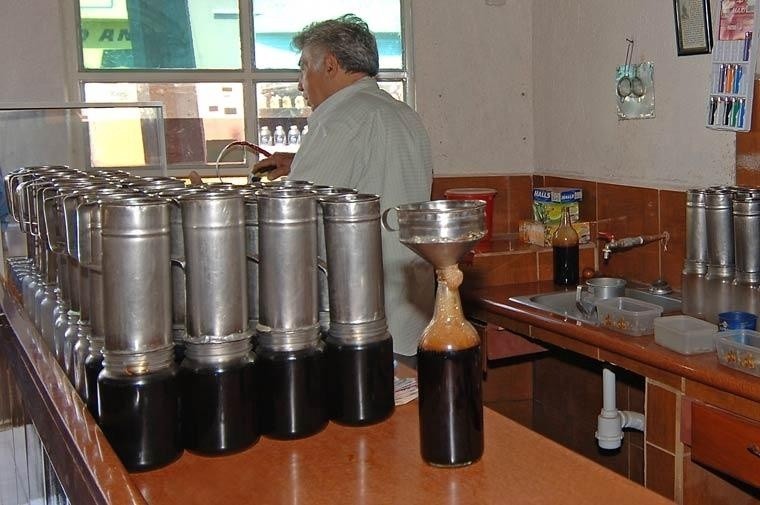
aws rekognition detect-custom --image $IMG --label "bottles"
[258,123,308,150]
[416,265,485,471]
[551,206,581,289]
[6,256,397,475]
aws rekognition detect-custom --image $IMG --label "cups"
[719,309,758,351]
[574,276,629,316]
[254,96,305,110]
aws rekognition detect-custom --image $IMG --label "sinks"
[509,288,683,327]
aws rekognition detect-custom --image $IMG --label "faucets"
[595,230,641,261]
[216,140,273,183]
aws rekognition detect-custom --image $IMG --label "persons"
[188,12,436,369]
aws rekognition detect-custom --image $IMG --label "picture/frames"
[673,0,714,56]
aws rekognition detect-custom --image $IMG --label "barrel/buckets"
[443,188,498,241]
